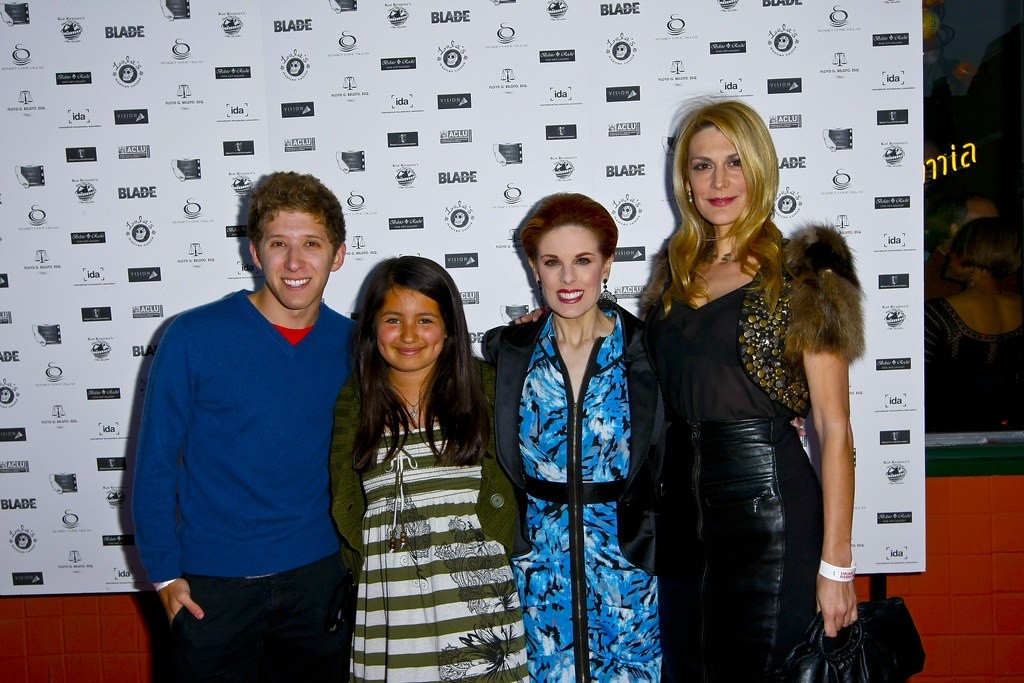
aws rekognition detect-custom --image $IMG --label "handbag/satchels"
[775,597,926,683]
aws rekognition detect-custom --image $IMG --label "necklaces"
[401,393,424,417]
[706,252,735,264]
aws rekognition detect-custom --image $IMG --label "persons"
[130,171,355,683]
[481,193,664,683]
[331,256,546,683]
[514,95,861,683]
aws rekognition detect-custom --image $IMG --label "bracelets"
[818,558,856,583]
